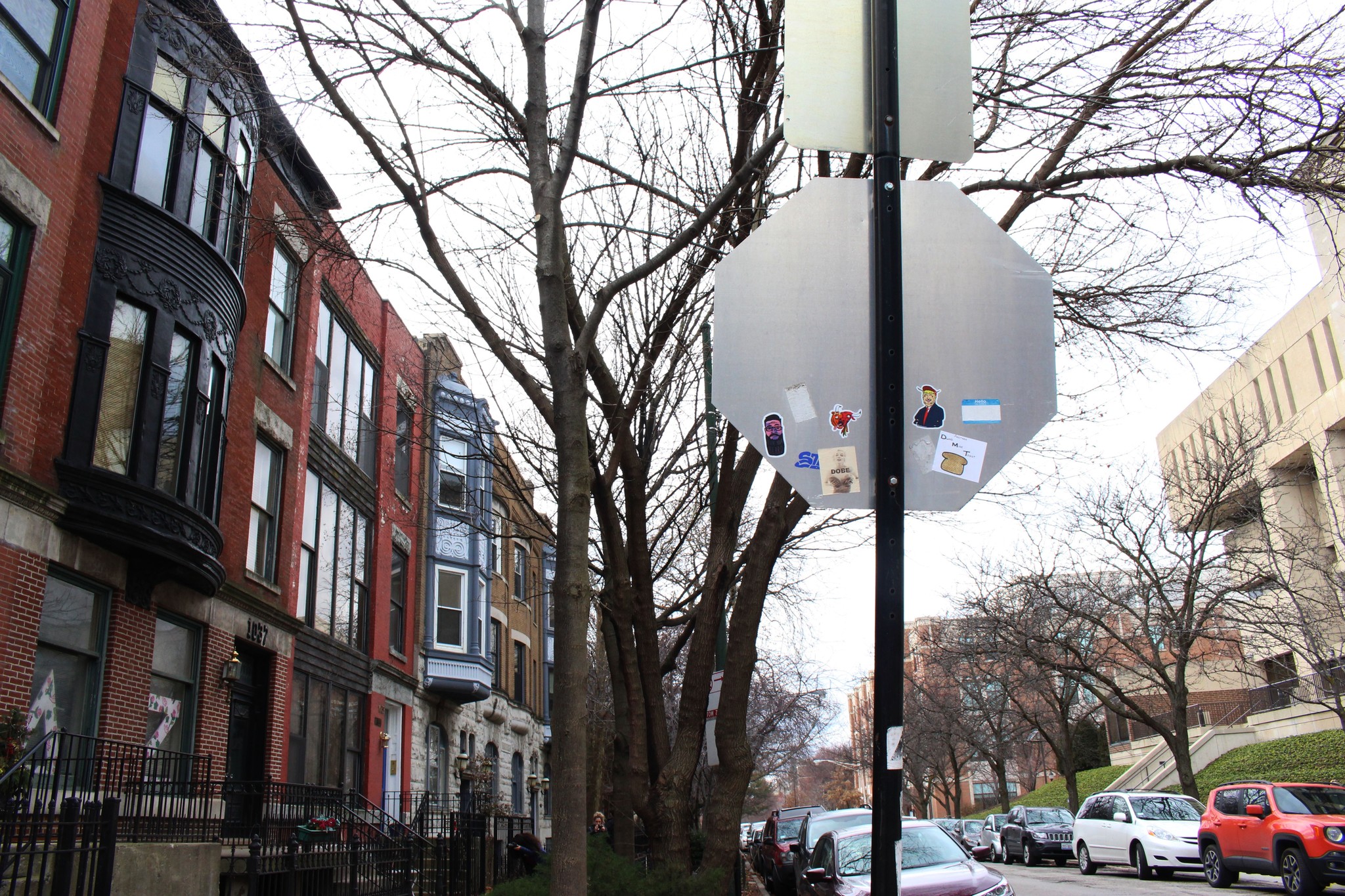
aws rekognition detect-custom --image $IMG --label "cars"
[795,819,1017,896]
[950,819,984,851]
[739,821,767,871]
[977,814,1007,864]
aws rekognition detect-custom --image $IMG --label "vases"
[296,825,337,843]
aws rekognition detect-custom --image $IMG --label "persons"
[511,832,548,877]
[824,449,858,493]
[588,810,617,844]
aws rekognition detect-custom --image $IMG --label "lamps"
[218,644,243,703]
[475,757,493,783]
[526,769,538,793]
[379,731,392,749]
[541,776,550,795]
[454,748,470,780]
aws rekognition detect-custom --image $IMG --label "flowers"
[307,813,341,833]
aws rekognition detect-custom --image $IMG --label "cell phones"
[598,825,602,828]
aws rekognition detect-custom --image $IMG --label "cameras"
[507,842,518,850]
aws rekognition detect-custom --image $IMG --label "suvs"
[789,804,873,875]
[761,805,826,890]
[1072,789,1207,881]
[1000,805,1076,867]
[1197,778,1345,896]
